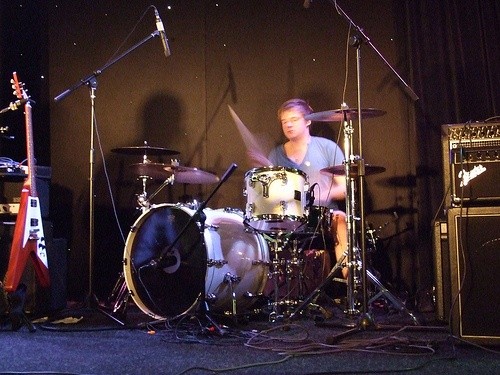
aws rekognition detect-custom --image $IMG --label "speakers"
[0,176,74,318]
[434,119,500,347]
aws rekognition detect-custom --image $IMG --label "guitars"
[2,71,52,294]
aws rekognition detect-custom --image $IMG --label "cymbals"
[126,163,220,184]
[318,163,387,178]
[109,146,182,157]
[304,107,389,122]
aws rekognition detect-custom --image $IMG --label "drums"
[241,165,313,233]
[285,204,335,254]
[122,203,271,321]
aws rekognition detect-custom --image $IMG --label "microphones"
[304,0,311,9]
[154,7,171,57]
[306,183,317,200]
[148,255,165,268]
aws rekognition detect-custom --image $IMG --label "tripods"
[282,0,451,344]
[48,30,248,336]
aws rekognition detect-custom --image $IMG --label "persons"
[268,99,348,276]
